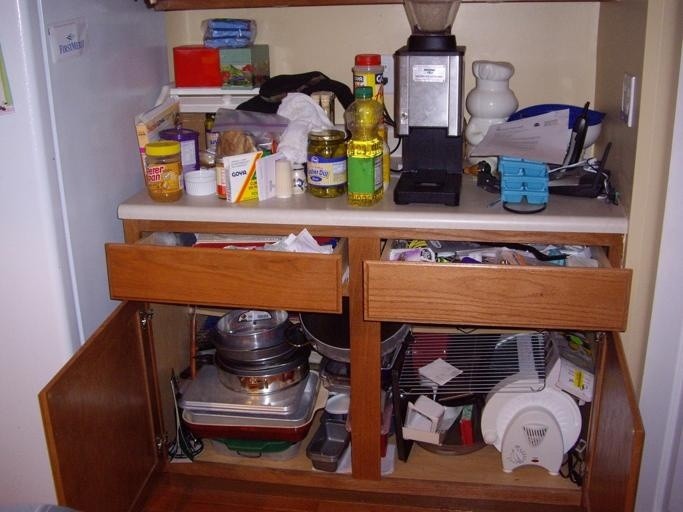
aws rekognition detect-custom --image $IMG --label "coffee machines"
[392,1,464,205]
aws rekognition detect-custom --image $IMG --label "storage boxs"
[221,44,270,89]
[546,334,594,407]
[171,43,219,89]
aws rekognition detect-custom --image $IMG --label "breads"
[216,130,257,158]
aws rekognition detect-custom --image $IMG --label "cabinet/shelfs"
[37,228,649,511]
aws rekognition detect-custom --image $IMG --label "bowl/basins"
[184,170,215,196]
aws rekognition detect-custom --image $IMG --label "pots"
[211,307,415,395]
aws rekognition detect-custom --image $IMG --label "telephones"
[549,101,613,199]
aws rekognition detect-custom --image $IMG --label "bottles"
[351,53,386,124]
[292,165,307,196]
[342,86,385,205]
[205,114,218,152]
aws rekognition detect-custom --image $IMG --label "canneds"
[305,129,349,197]
[203,112,220,152]
[142,140,184,204]
[215,158,227,200]
